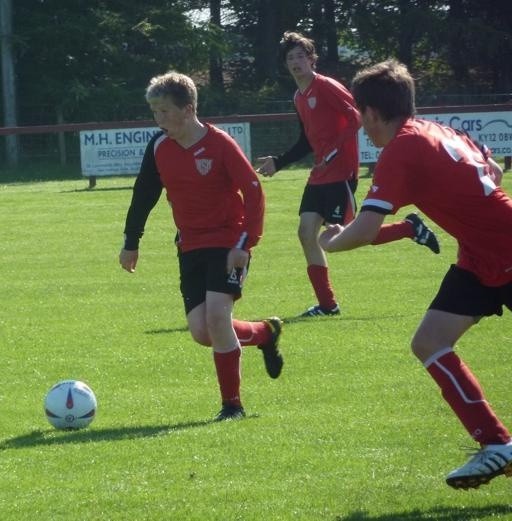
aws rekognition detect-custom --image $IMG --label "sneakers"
[297,303,341,318]
[444,435,512,491]
[211,402,247,424]
[256,316,285,379]
[403,212,441,255]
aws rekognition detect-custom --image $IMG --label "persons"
[119,72,284,422]
[317,60,512,490]
[256,32,440,318]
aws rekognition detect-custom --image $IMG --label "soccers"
[45,380,96,430]
